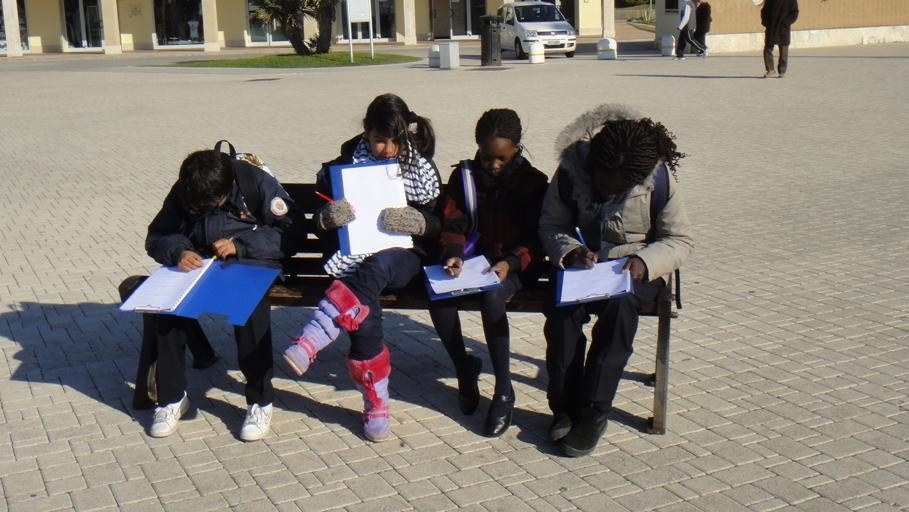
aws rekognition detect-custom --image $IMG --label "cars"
[249,2,263,21]
[495,1,577,60]
[0,9,25,39]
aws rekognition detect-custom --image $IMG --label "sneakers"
[764,70,776,77]
[240,403,273,441]
[151,390,191,438]
[672,46,709,60]
[778,73,784,78]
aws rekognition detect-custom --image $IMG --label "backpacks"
[212,140,288,220]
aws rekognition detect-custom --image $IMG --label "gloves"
[383,204,426,235]
[318,200,356,231]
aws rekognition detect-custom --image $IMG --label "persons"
[427,108,550,436]
[693,0,712,56]
[281,92,445,442]
[145,149,306,441]
[760,0,799,78]
[671,0,709,60]
[538,102,695,457]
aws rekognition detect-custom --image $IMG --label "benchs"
[118,180,683,435]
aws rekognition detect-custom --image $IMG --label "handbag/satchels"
[462,229,482,258]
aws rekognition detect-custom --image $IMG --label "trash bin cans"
[479,14,503,66]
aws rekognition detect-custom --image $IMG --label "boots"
[282,280,370,376]
[560,397,611,456]
[547,385,577,441]
[346,345,391,442]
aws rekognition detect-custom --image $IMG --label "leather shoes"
[484,386,515,436]
[457,357,482,415]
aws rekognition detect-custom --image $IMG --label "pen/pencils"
[443,266,459,270]
[212,237,234,260]
[315,191,334,202]
[576,227,597,267]
